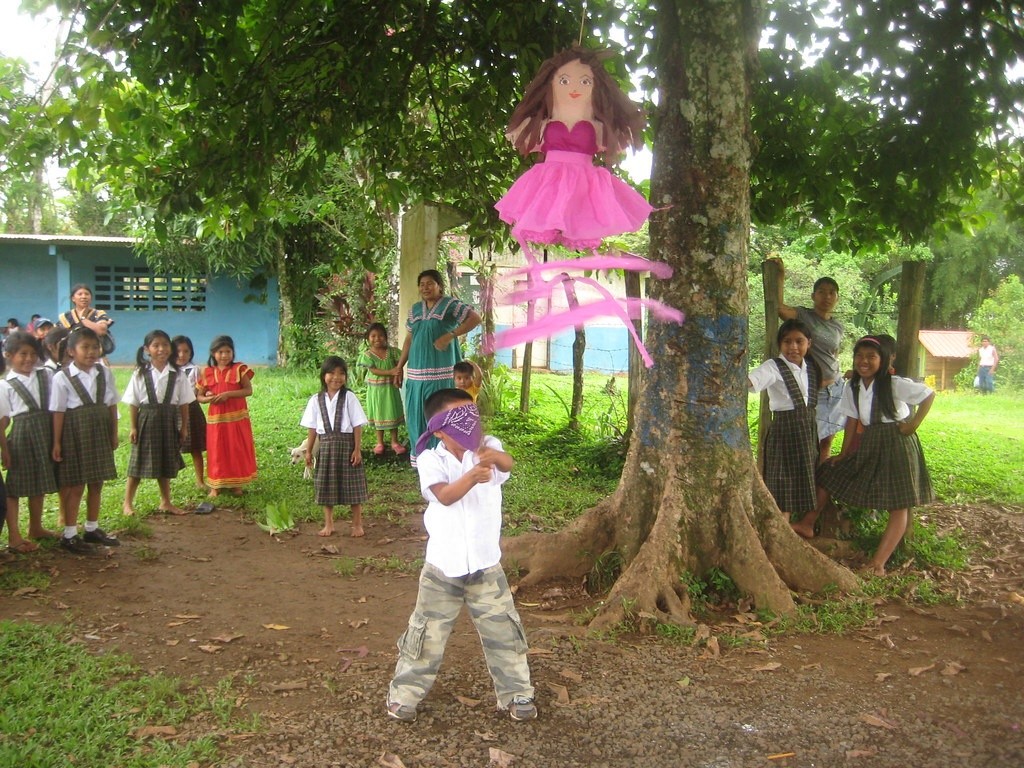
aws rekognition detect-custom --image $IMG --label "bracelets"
[450,331,456,336]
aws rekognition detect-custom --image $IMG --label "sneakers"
[386,691,416,724]
[509,701,537,721]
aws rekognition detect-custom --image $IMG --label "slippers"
[195,502,215,514]
[374,446,385,454]
[393,443,406,454]
[8,538,37,552]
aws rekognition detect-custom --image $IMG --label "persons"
[0,314,118,564]
[746,318,824,522]
[357,323,405,454]
[770,257,849,472]
[384,388,537,722]
[196,335,258,497]
[393,269,483,466]
[121,330,196,515]
[977,337,999,394]
[789,335,935,577]
[300,356,368,537]
[171,335,212,487]
[54,283,113,368]
[492,49,654,250]
[453,358,483,402]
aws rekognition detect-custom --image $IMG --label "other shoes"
[83,528,118,545]
[61,531,92,553]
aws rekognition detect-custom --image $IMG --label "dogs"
[288,437,320,480]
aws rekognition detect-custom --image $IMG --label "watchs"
[80,317,85,320]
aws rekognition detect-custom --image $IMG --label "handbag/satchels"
[94,311,114,353]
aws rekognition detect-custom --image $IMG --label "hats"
[34,318,53,330]
